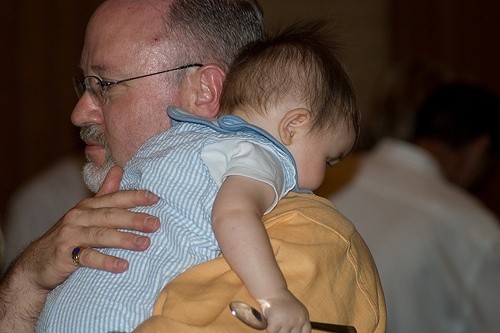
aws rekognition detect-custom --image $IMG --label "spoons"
[229,301,357,333]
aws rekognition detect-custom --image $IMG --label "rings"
[72,245,89,267]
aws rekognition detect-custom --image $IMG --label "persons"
[312,77,500,333]
[0,0,387,333]
[34,19,362,333]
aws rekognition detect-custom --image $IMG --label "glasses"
[72,64,206,107]
